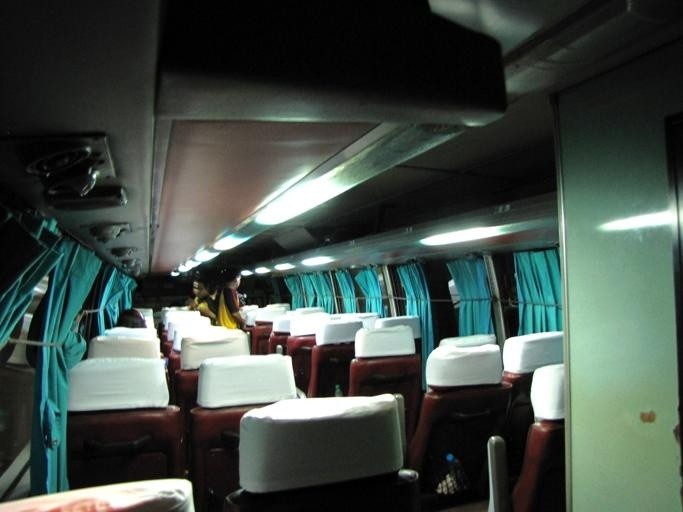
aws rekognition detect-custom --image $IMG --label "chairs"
[1,478,195,510]
[63,356,185,483]
[3,302,422,400]
[243,393,410,511]
[438,332,497,351]
[191,352,297,511]
[412,344,503,512]
[501,328,565,489]
[505,361,565,511]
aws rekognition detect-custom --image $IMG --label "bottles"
[445,453,468,489]
[334,383,342,399]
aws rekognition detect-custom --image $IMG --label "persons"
[116,308,148,330]
[214,266,247,332]
[186,277,218,326]
